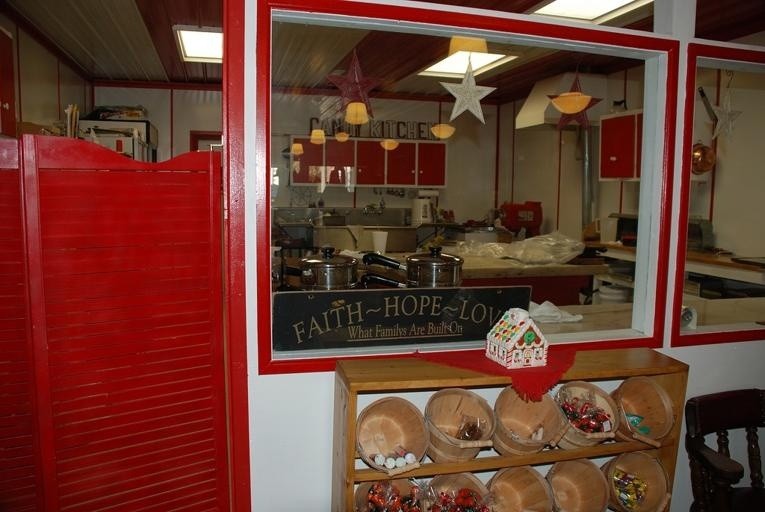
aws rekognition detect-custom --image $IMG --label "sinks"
[311,224,418,253]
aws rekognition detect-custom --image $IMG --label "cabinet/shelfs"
[290,135,324,186]
[597,109,643,182]
[591,242,765,313]
[324,136,386,187]
[385,139,447,188]
[333,347,690,512]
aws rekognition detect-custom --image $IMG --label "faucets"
[364,198,387,215]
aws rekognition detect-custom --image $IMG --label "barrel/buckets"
[546,459,610,512]
[486,465,554,512]
[425,388,497,465]
[556,380,619,450]
[600,452,672,512]
[490,385,563,457]
[610,377,676,448]
[353,397,430,477]
[427,473,486,511]
[355,479,426,512]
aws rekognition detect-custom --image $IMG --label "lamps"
[431,124,457,139]
[447,36,487,58]
[344,101,370,125]
[334,132,349,142]
[172,25,223,64]
[550,91,591,115]
[377,139,398,150]
[290,143,305,156]
[310,129,326,145]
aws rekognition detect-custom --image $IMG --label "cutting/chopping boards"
[459,251,609,279]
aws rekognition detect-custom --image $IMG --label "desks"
[359,251,609,306]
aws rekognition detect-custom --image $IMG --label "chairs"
[684,388,765,512]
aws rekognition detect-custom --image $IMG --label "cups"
[372,231,388,254]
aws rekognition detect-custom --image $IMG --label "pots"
[362,245,465,289]
[298,246,359,291]
